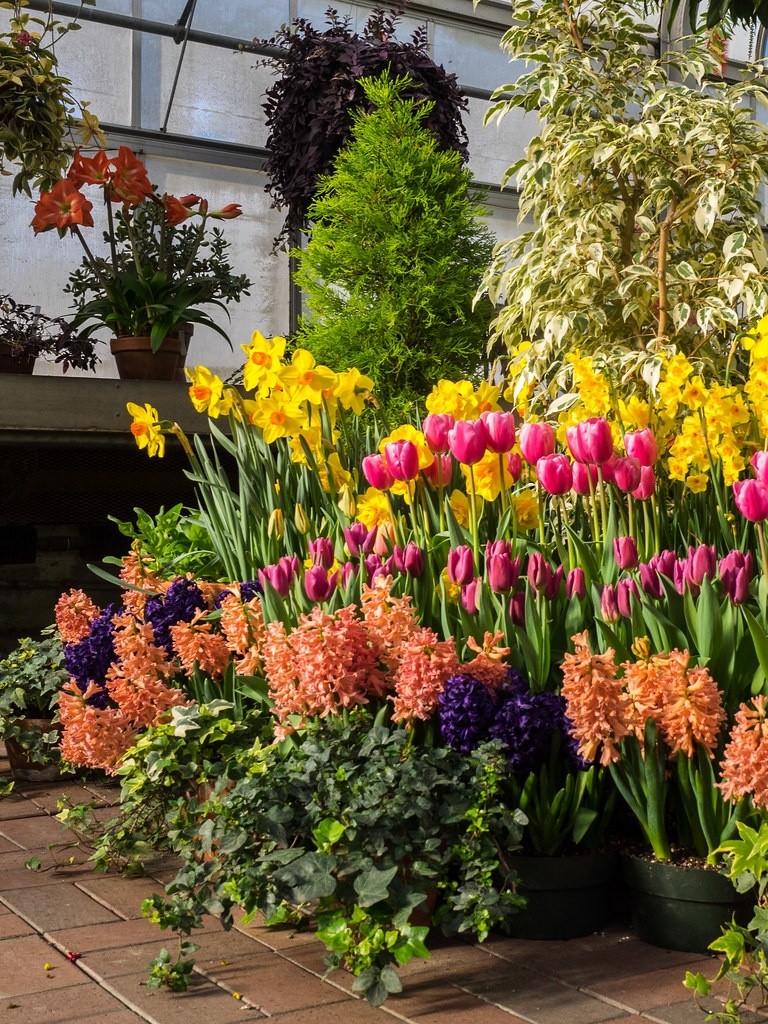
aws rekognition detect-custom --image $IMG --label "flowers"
[27,144,255,332]
[52,332,768,868]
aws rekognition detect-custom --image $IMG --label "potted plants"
[0,293,107,375]
[334,755,483,932]
[0,636,64,784]
[111,718,272,862]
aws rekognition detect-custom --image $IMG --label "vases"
[631,848,755,952]
[175,322,194,378]
[507,845,617,941]
[110,337,182,377]
